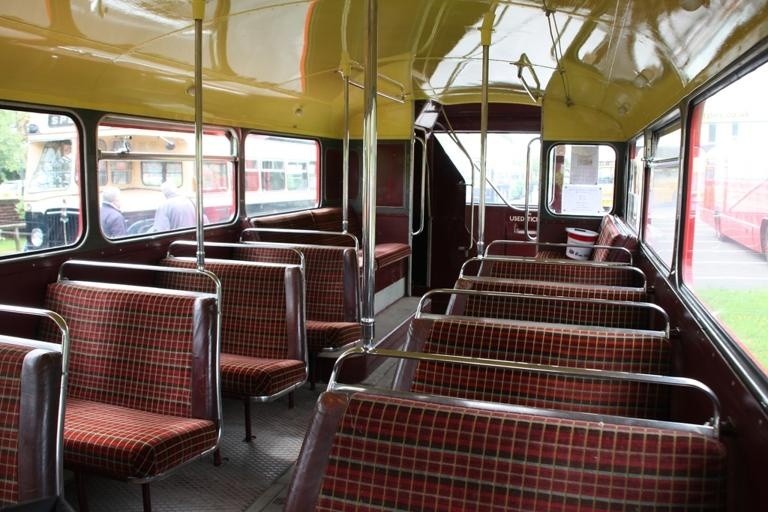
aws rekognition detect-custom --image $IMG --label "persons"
[100,187,128,236]
[154,179,210,232]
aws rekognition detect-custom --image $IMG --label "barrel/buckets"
[565,226,599,260]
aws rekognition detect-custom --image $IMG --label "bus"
[23,111,317,252]
[691,60,767,263]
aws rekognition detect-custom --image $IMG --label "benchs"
[444,255,650,332]
[475,214,644,284]
[283,347,736,512]
[238,226,361,390]
[390,289,677,421]
[246,206,411,315]
[0,303,77,511]
[42,258,226,512]
[158,239,310,443]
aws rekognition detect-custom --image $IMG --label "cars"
[464,154,536,202]
[599,168,677,211]
